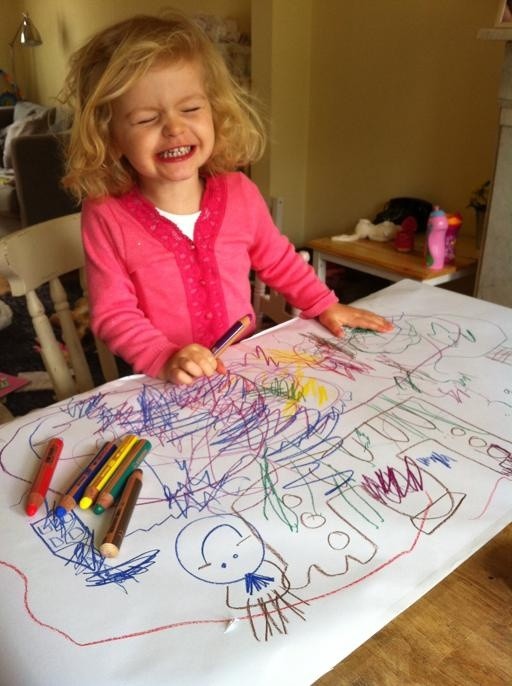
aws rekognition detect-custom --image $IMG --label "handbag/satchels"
[370,197,432,234]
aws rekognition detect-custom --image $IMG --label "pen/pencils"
[210,314,253,360]
[26,435,152,558]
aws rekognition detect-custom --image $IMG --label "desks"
[0,278,512,686]
[306,232,480,301]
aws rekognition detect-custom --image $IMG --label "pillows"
[3,107,56,169]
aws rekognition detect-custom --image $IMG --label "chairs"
[0,103,77,228]
[0,211,125,402]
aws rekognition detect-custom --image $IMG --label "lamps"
[8,10,43,102]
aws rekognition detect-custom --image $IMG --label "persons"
[53,9,395,388]
